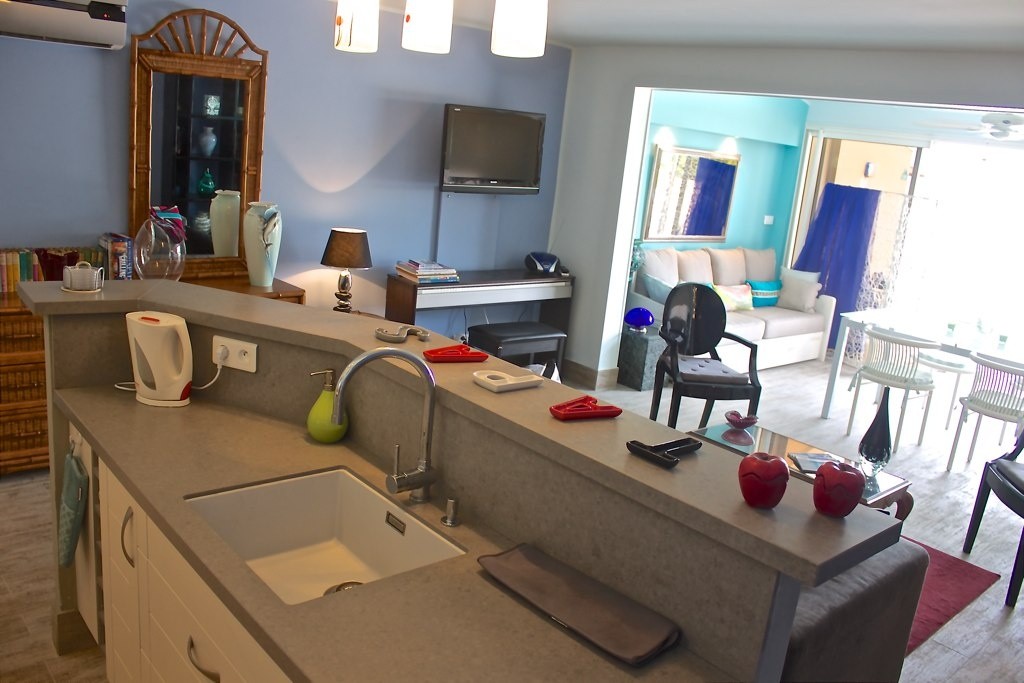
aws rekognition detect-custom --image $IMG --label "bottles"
[196,171,216,198]
[208,189,241,257]
[134,219,187,283]
[197,126,217,157]
[242,201,283,288]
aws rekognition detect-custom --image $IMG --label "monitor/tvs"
[440,103,546,195]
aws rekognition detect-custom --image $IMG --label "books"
[0,247,104,292]
[788,452,841,472]
[396,259,459,283]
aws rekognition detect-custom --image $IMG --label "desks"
[385,268,576,382]
[821,309,1024,418]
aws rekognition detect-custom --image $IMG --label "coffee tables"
[685,422,914,522]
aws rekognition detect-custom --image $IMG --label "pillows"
[635,246,822,312]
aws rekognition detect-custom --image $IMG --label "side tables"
[617,326,670,392]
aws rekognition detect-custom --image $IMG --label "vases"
[243,201,282,286]
[192,127,241,256]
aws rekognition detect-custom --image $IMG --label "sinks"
[180,464,469,608]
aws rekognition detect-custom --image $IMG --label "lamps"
[491,0,548,58]
[319,227,373,313]
[401,0,454,55]
[334,0,380,53]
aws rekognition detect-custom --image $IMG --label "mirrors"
[130,9,269,279]
[641,144,742,244]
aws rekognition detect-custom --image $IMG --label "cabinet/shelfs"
[0,276,306,683]
[160,70,244,255]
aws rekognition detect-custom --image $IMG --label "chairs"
[648,287,1024,609]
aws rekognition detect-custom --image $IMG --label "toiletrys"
[307,369,349,443]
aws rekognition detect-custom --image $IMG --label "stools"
[467,322,568,390]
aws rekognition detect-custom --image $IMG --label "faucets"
[327,348,441,504]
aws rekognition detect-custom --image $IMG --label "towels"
[476,542,684,668]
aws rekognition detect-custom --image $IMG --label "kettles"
[125,309,194,407]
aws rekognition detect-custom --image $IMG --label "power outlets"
[213,335,258,373]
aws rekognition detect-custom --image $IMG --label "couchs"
[618,247,837,375]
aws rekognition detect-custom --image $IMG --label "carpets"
[904,535,1002,657]
